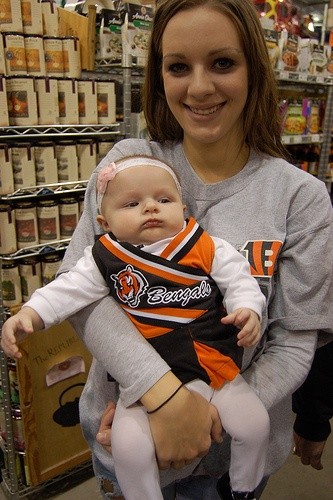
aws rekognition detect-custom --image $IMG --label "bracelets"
[146,383,183,414]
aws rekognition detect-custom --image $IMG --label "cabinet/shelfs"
[0,2,333,500]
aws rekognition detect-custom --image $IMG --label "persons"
[52,0,333,500]
[290,181,333,470]
[0,152,271,500]
